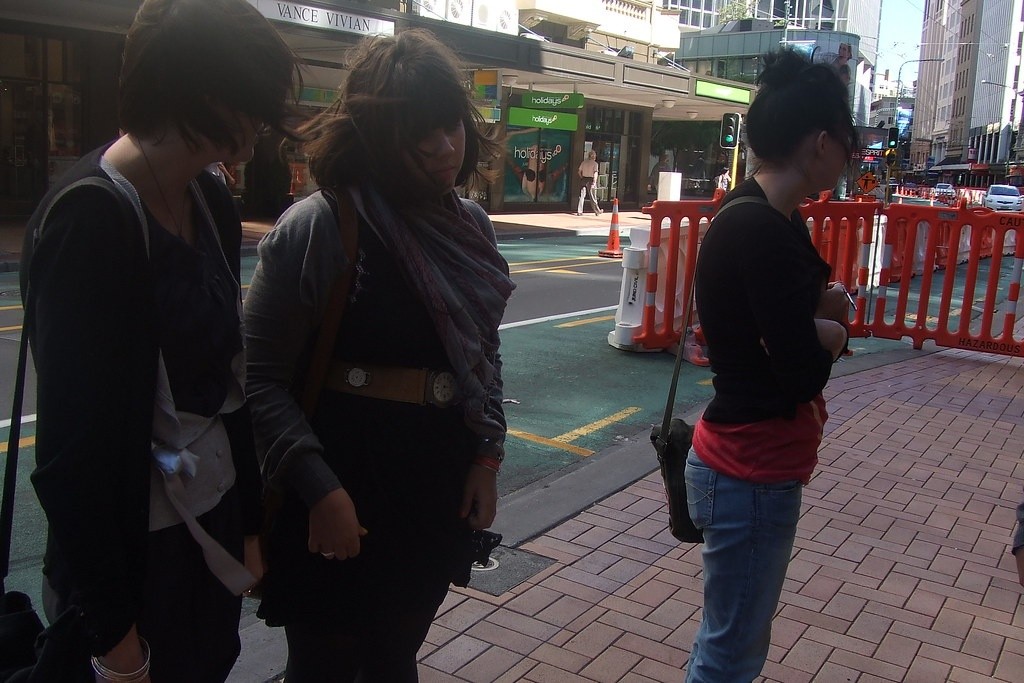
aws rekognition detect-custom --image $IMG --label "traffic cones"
[929,195,934,207]
[898,197,903,203]
[895,185,916,197]
[597,198,622,258]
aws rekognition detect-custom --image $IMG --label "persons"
[718,167,732,192]
[649,154,671,193]
[887,148,895,166]
[241,28,516,683]
[576,151,604,216]
[205,162,235,185]
[830,41,852,86]
[18,0,292,683]
[684,47,856,683]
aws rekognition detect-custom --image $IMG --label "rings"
[321,551,335,556]
[248,589,251,593]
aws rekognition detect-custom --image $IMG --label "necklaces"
[135,135,190,238]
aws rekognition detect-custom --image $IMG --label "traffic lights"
[888,128,899,149]
[885,151,897,167]
[719,113,740,149]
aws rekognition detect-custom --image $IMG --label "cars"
[934,183,956,199]
[880,178,898,189]
[900,182,919,193]
[982,185,1024,211]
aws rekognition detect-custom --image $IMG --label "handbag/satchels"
[650,418,706,544]
[0,591,96,683]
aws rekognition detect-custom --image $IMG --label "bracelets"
[478,456,501,476]
[91,636,151,683]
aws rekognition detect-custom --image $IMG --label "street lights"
[981,79,1017,185]
[885,59,943,203]
